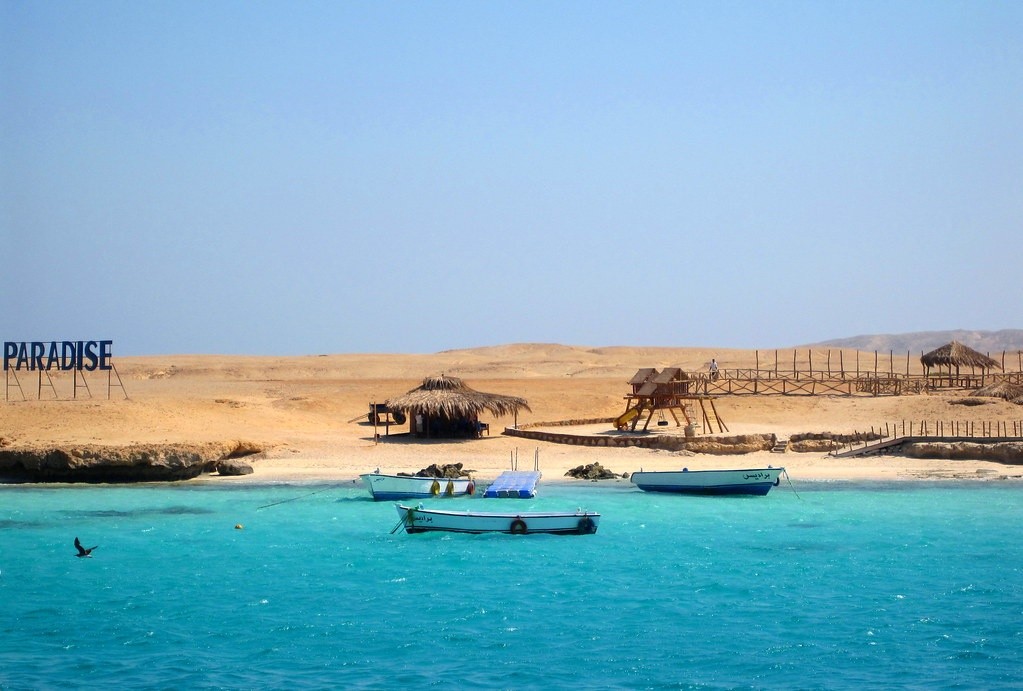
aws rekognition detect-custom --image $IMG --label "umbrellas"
[920,341,1001,386]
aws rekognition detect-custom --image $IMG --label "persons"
[709,359,719,382]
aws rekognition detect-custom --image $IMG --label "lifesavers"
[510,520,527,534]
[431,481,474,495]
[772,477,780,488]
[577,517,595,535]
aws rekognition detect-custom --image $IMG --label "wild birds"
[74,537,98,558]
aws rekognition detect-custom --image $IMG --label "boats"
[359,473,475,502]
[630,465,784,496]
[395,502,601,535]
